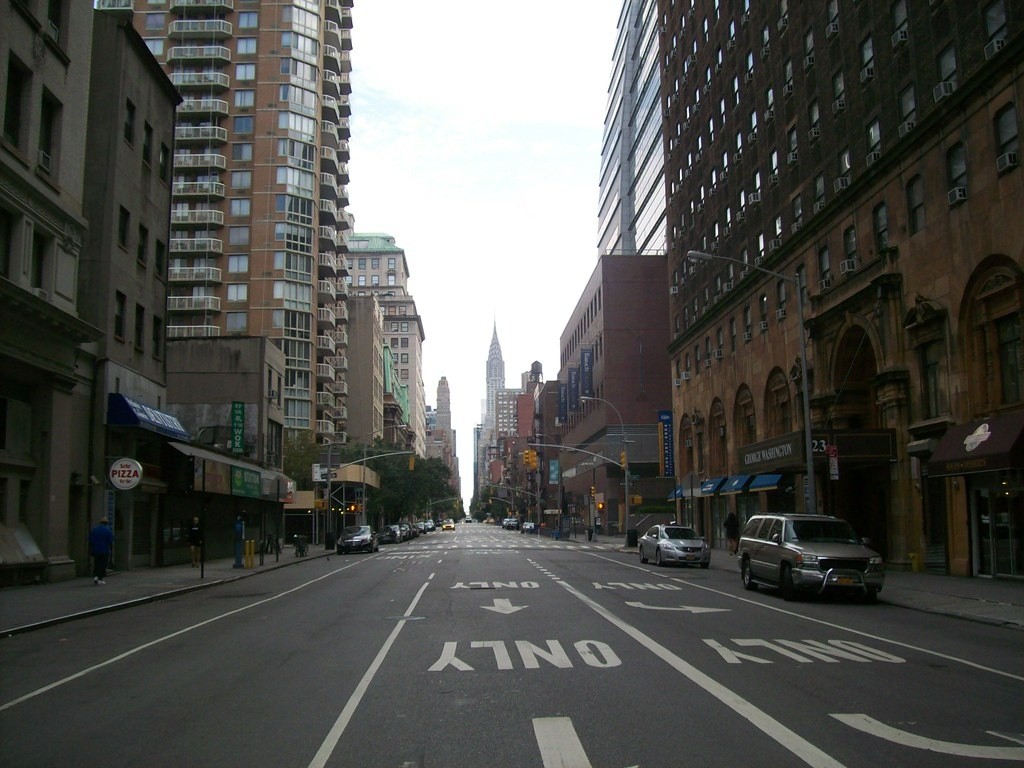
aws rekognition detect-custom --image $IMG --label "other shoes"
[192,562,200,568]
[730,553,733,555]
[93,576,106,585]
[733,550,737,554]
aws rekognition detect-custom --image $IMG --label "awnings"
[749,475,782,492]
[719,475,754,495]
[928,412,1024,477]
[667,486,685,502]
[701,477,727,497]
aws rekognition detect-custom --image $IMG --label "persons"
[723,512,739,556]
[186,516,202,568]
[90,517,114,585]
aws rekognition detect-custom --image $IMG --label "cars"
[502,519,519,530]
[638,525,711,568]
[465,516,472,522]
[522,522,534,533]
[442,520,454,530]
[376,520,435,544]
[335,525,379,554]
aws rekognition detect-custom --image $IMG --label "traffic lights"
[590,486,595,497]
[349,504,356,511]
[523,450,529,464]
[597,502,605,510]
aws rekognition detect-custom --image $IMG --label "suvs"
[737,513,885,599]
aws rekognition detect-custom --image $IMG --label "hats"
[100,517,108,522]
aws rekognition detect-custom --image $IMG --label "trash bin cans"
[626,529,638,547]
[324,532,336,550]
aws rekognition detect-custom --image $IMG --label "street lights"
[499,482,513,513]
[535,433,562,529]
[581,396,629,546]
[687,250,818,514]
[362,425,407,525]
